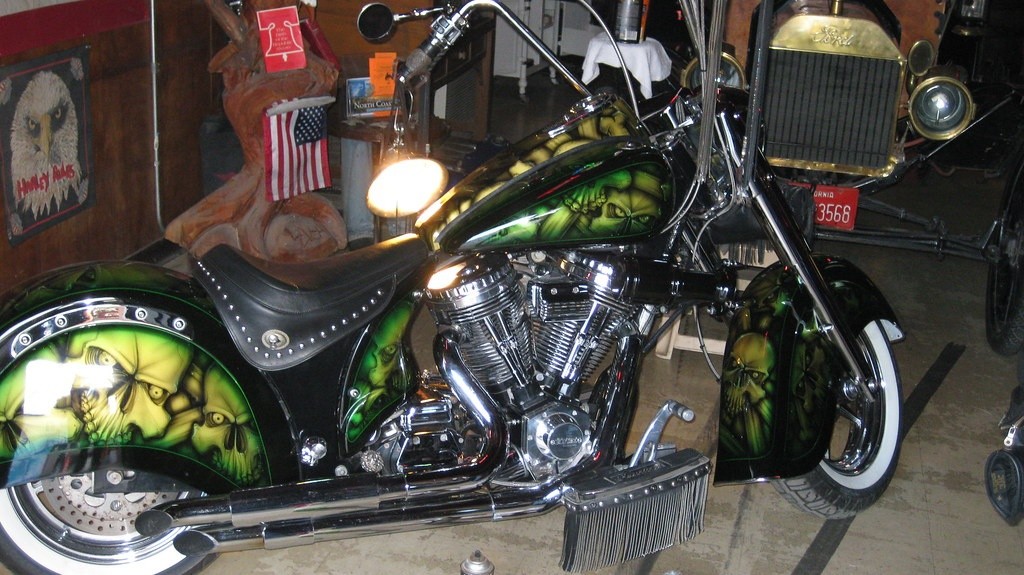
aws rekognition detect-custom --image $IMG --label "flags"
[263,94,336,201]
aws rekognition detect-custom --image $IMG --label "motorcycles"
[0,0,975,575]
